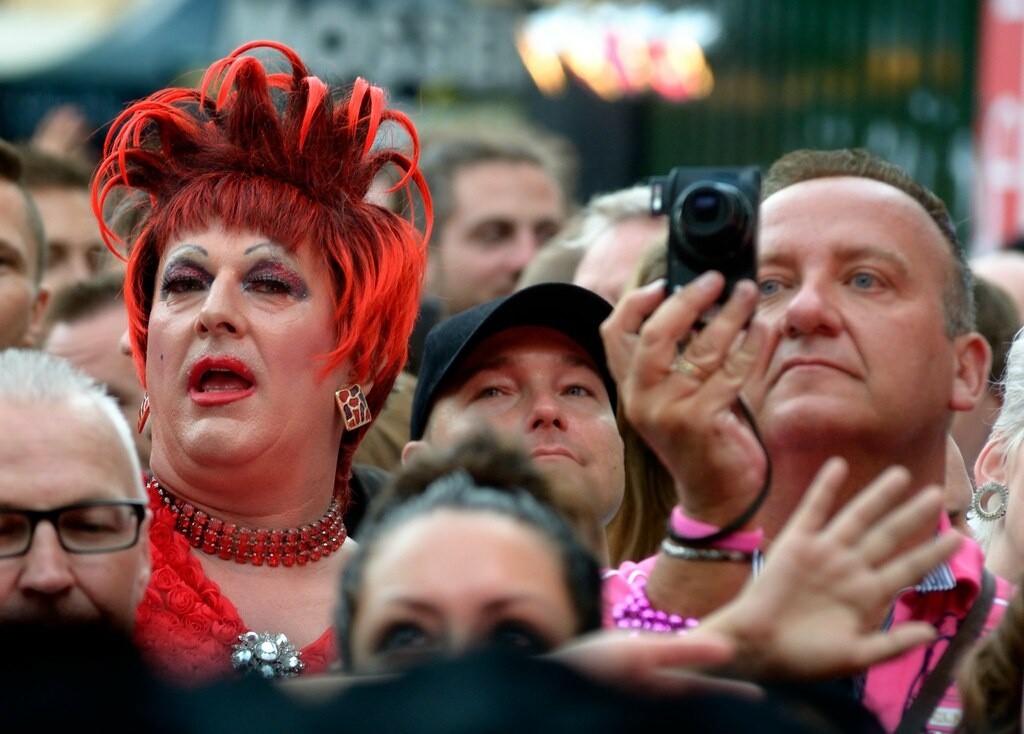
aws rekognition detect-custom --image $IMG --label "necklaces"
[147,477,347,567]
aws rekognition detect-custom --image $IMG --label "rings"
[671,356,708,381]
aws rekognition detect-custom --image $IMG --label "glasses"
[0,498,146,559]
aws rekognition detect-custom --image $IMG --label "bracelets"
[660,539,753,562]
[669,505,762,552]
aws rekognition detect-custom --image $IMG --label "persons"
[0,38,1024,734]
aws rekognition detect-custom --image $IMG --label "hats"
[409,281,624,439]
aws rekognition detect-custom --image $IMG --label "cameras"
[649,165,761,332]
